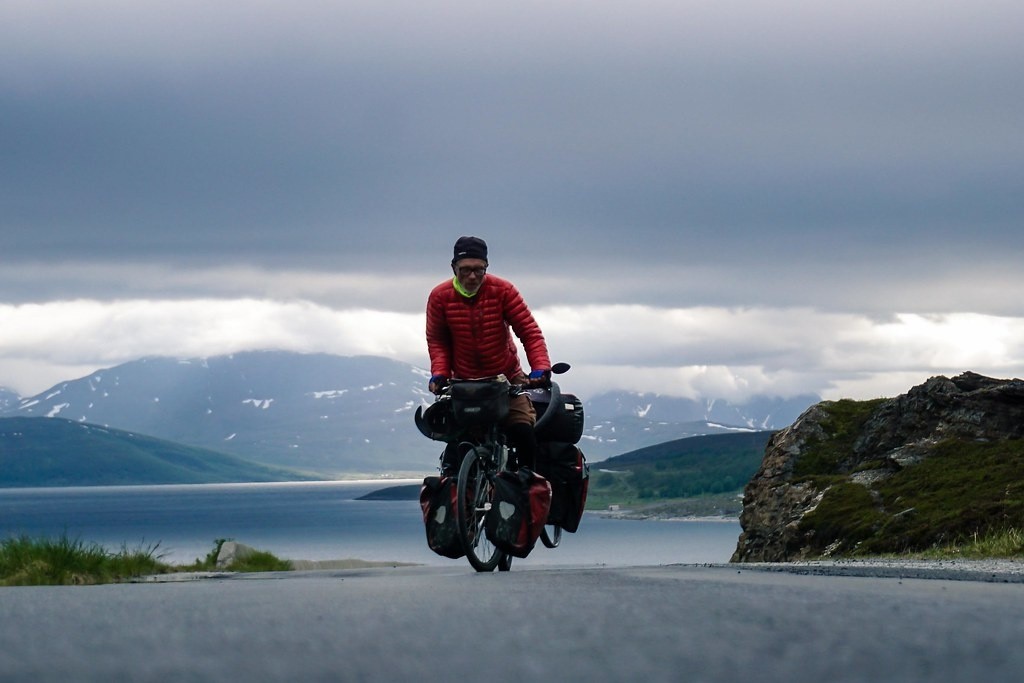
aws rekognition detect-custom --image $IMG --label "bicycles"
[436,362,553,574]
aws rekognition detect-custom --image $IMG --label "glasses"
[456,264,487,277]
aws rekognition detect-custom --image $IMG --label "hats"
[453,236,490,261]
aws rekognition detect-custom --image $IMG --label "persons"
[425,236,553,482]
